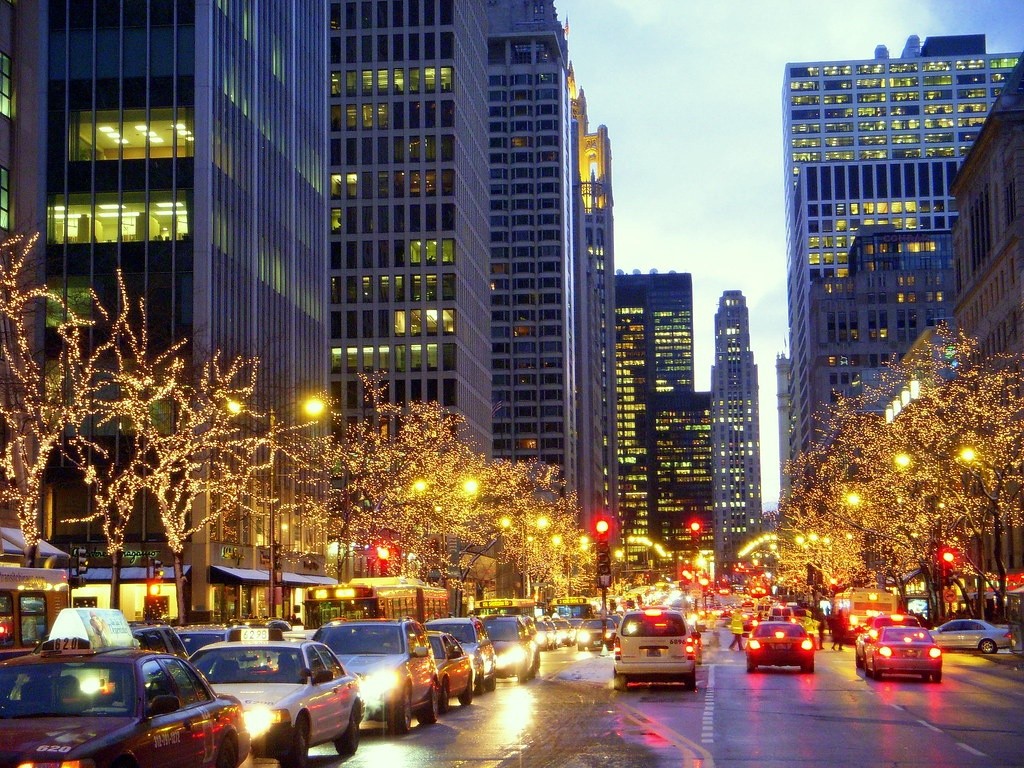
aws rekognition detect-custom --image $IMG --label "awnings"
[209,566,341,586]
[0,527,70,559]
[58,565,191,581]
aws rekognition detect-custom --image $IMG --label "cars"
[231,616,292,646]
[0,607,250,767]
[134,625,188,656]
[427,612,626,714]
[190,639,368,768]
[178,624,251,655]
[852,615,942,684]
[612,604,701,692]
[928,618,1011,654]
[738,596,815,674]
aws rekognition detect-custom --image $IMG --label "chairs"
[211,660,240,682]
[18,679,57,708]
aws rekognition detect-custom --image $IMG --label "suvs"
[308,616,440,738]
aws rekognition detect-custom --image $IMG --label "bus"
[547,592,595,621]
[833,586,897,641]
[471,596,538,623]
[300,576,454,626]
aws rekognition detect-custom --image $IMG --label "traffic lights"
[814,563,824,585]
[596,540,612,588]
[689,519,702,557]
[807,565,814,586]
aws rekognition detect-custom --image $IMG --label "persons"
[728,609,746,651]
[815,607,848,652]
[908,600,1019,629]
[52,675,83,704]
